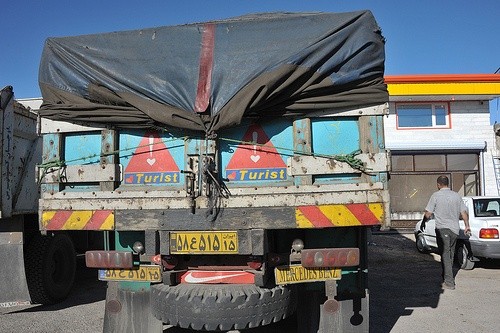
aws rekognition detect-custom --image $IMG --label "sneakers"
[441,282,455,290]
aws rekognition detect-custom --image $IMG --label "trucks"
[32,8,391,333]
[0,85,103,315]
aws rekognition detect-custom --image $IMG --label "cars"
[414,195,500,270]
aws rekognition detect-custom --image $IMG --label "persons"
[420,176,472,290]
